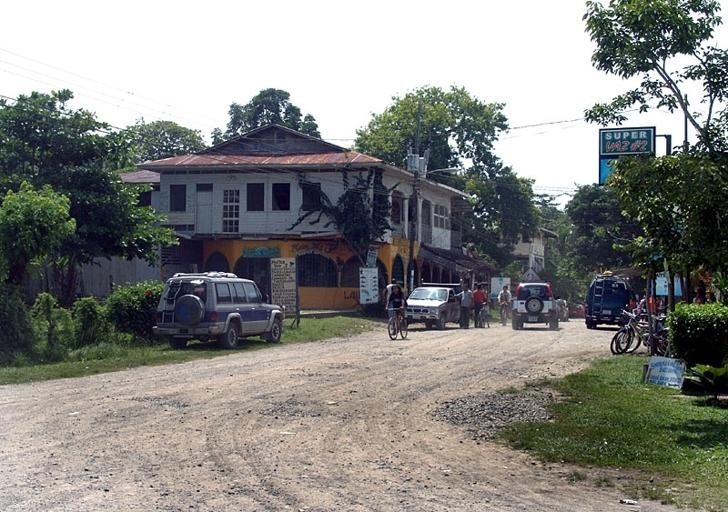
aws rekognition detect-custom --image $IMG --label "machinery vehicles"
[582,274,640,331]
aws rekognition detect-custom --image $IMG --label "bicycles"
[607,306,675,359]
[500,300,510,327]
[479,299,489,330]
[383,308,413,339]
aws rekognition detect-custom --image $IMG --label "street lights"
[405,167,463,293]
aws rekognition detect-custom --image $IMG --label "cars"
[553,298,588,322]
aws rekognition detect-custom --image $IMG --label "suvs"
[509,281,561,332]
[148,269,289,347]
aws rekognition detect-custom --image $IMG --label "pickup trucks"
[402,285,464,331]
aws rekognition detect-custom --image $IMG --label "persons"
[454,283,487,329]
[383,279,405,330]
[497,286,511,322]
